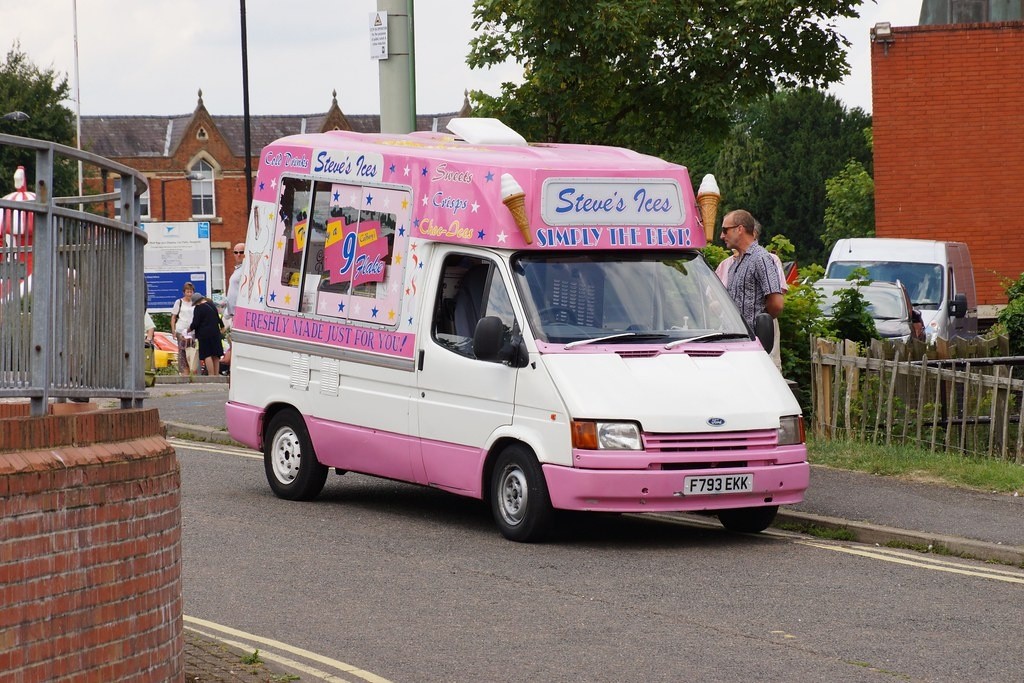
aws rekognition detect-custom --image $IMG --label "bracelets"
[147,337,151,341]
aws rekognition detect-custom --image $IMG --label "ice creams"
[500,173,533,245]
[697,173,720,241]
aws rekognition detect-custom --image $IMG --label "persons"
[720,210,784,333]
[706,219,788,372]
[187,293,224,375]
[170,281,196,375]
[227,243,245,316]
[144,312,156,343]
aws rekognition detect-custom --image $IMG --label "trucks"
[226,119,809,542]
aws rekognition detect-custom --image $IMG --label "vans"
[825,237,979,347]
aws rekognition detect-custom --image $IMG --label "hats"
[191,293,204,306]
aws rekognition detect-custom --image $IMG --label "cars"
[805,277,927,344]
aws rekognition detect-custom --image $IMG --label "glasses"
[722,225,747,234]
[234,251,245,254]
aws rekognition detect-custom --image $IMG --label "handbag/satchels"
[186,332,198,371]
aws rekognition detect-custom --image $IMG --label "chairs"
[450,263,489,356]
